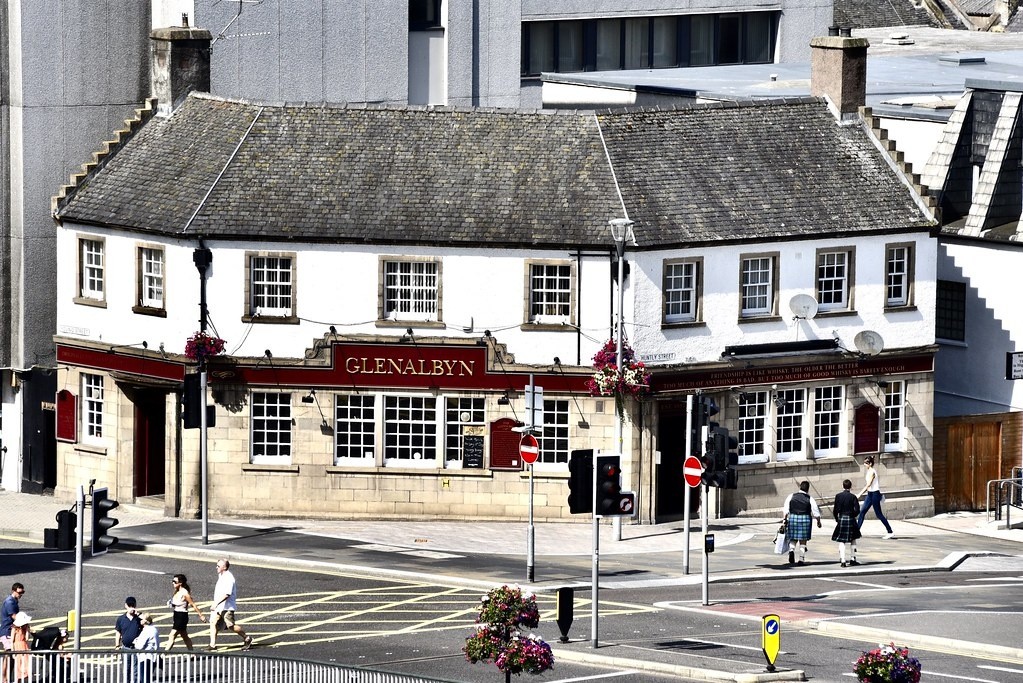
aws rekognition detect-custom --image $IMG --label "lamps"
[546,355,560,374]
[400,326,415,344]
[477,330,492,346]
[866,378,889,388]
[301,389,315,404]
[498,395,509,407]
[108,341,147,356]
[732,389,752,401]
[255,349,272,367]
[329,326,339,342]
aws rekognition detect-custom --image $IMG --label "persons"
[831,480,862,566]
[134,614,159,683]
[11,612,33,683]
[160,574,206,659]
[0,583,25,683]
[783,480,821,565]
[115,597,144,683]
[855,456,894,539]
[200,559,252,651]
[58,631,71,661]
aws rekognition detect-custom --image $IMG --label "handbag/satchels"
[773,522,789,555]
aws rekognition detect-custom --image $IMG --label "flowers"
[853,641,922,683]
[186,331,225,362]
[586,338,651,424]
[460,585,555,675]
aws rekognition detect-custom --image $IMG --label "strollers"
[28,627,90,683]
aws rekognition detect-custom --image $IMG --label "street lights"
[608,218,635,381]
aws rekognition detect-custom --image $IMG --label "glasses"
[172,580,177,584]
[13,590,26,595]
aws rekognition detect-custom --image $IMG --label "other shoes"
[882,532,894,540]
[787,551,796,563]
[850,559,860,566]
[798,558,805,563]
[241,636,253,651]
[201,645,218,651]
[841,562,845,567]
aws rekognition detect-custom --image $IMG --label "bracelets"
[116,645,119,647]
[138,613,142,616]
[784,519,786,520]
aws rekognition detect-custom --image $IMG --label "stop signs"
[682,456,702,488]
[518,435,539,463]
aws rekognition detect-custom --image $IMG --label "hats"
[138,613,153,624]
[126,597,136,607]
[13,611,32,627]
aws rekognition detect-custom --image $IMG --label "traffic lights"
[91,487,119,557]
[701,428,738,489]
[596,455,621,515]
[568,449,592,514]
